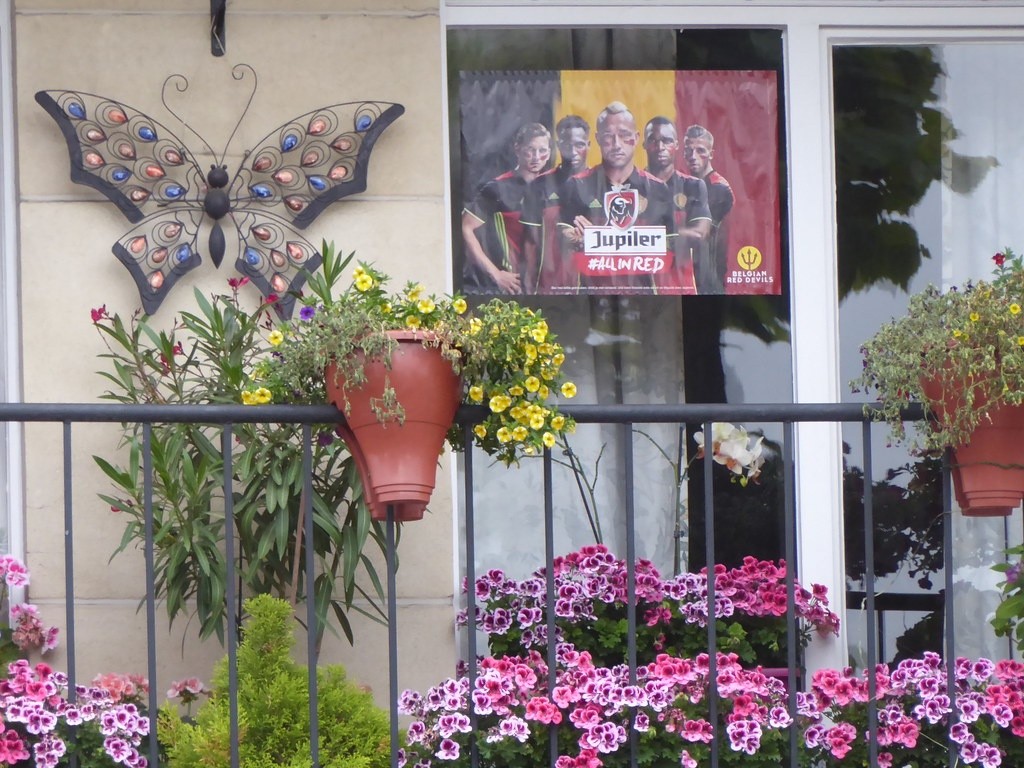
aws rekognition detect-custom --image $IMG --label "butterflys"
[33,63,406,334]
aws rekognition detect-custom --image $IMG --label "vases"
[744,668,800,694]
[327,395,429,522]
[930,420,1013,517]
[913,328,1024,508]
[322,331,465,502]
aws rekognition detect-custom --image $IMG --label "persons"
[461,102,736,295]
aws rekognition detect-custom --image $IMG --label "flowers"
[543,421,766,579]
[239,261,578,467]
[847,246,1024,461]
